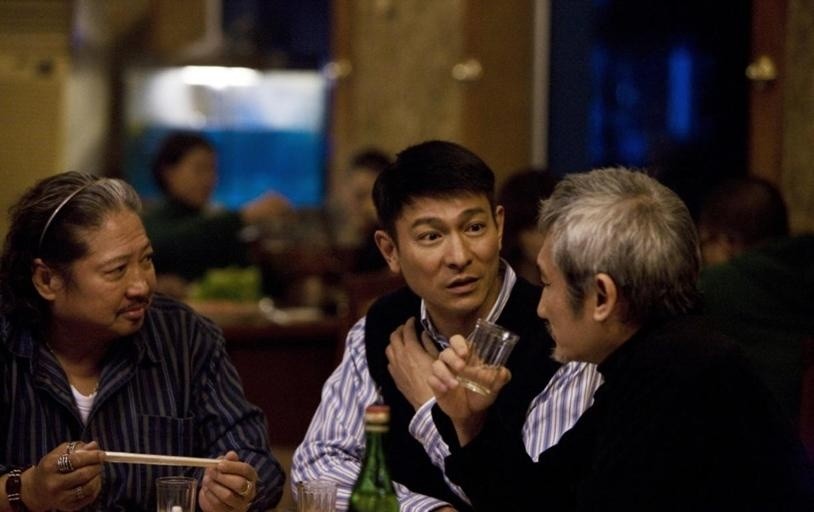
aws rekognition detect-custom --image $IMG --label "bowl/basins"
[193,303,265,331]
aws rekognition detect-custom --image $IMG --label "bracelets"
[5,469,30,511]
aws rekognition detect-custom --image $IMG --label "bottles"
[349,407,397,510]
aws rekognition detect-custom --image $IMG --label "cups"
[295,474,338,510]
[457,317,520,395]
[153,473,197,510]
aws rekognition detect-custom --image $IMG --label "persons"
[136,129,295,305]
[0,171,287,511]
[494,168,814,471]
[291,140,605,511]
[427,166,814,511]
[294,150,391,277]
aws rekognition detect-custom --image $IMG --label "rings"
[76,486,85,499]
[57,453,75,473]
[238,478,253,497]
[66,441,80,453]
